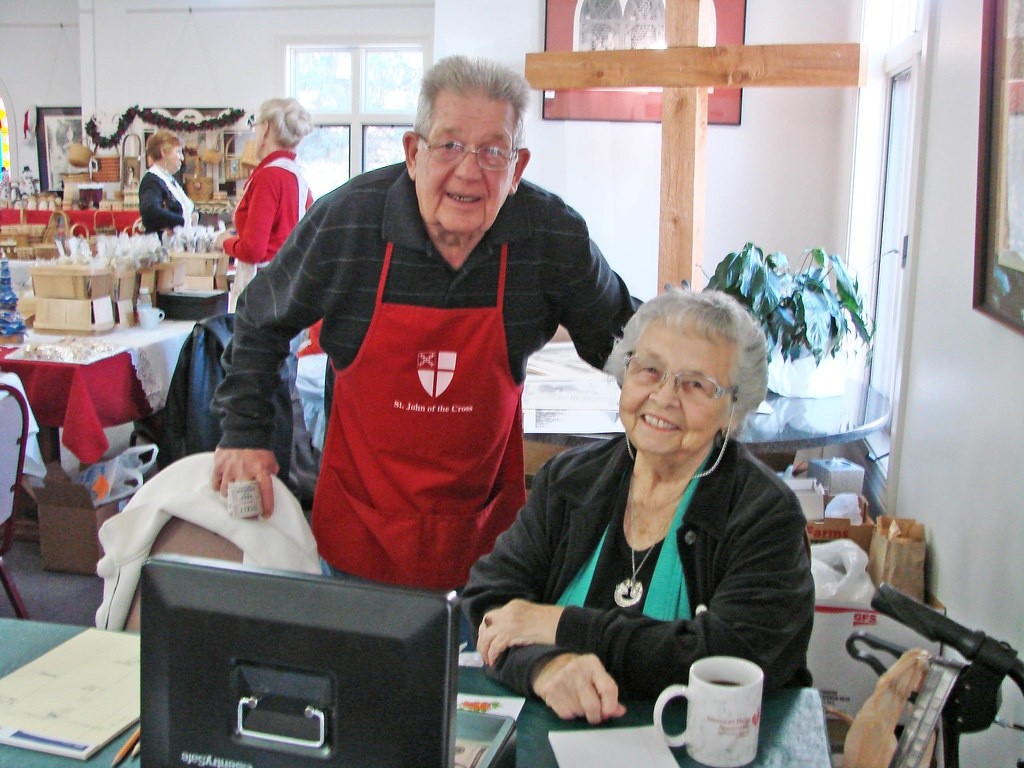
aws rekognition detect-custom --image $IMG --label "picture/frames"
[34,105,83,199]
[971,0,1024,338]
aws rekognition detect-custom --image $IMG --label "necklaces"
[614,472,675,607]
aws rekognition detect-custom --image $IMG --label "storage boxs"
[20,457,147,575]
[785,479,876,555]
[157,288,228,320]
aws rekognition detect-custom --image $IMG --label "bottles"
[136,287,152,321]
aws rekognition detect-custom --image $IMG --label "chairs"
[113,450,323,636]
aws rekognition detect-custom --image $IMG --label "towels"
[93,449,323,633]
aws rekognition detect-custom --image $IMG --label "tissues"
[805,457,865,496]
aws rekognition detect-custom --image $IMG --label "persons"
[210,54,647,590]
[139,133,201,244]
[215,98,320,358]
[50,123,74,190]
[463,288,818,721]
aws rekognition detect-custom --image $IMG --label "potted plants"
[706,239,874,397]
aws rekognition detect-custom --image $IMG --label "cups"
[138,306,165,329]
[652,657,765,768]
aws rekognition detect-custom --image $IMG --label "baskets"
[66,143,93,169]
[184,158,215,203]
[123,190,140,210]
[88,156,121,183]
[58,172,89,187]
[0,210,117,261]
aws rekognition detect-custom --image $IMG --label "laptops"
[138,558,516,768]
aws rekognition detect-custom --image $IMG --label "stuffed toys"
[0,168,10,202]
[21,166,39,195]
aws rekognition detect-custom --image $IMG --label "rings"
[484,616,492,628]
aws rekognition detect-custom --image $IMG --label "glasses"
[415,129,516,172]
[622,351,737,405]
[250,121,262,130]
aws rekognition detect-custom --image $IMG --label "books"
[0,627,141,759]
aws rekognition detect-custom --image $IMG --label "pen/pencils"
[130,740,141,758]
[110,726,141,768]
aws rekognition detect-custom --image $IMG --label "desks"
[0,618,831,768]
[510,340,888,476]
[0,316,202,470]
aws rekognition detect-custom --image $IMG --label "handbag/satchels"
[74,442,159,505]
[802,537,929,720]
